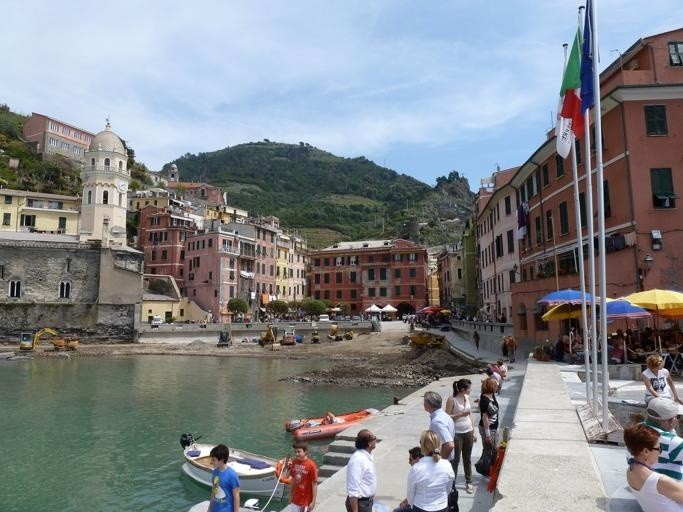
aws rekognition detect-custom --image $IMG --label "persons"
[500,312,506,333]
[288,440,317,512]
[624,425,683,511]
[445,379,477,494]
[486,331,517,403]
[345,429,377,512]
[551,326,683,364]
[475,379,499,478]
[424,391,455,472]
[393,447,424,512]
[642,355,683,408]
[207,445,241,512]
[473,331,480,350]
[407,430,455,512]
[627,398,683,482]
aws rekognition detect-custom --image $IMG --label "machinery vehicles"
[241,321,355,344]
[15,327,80,353]
[259,323,281,351]
[216,323,233,348]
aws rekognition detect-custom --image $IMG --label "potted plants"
[535,345,551,361]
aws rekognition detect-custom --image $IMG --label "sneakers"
[466,483,473,493]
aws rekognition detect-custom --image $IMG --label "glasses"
[371,436,376,441]
[675,415,680,419]
[652,446,661,453]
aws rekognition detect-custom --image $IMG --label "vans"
[150,315,163,329]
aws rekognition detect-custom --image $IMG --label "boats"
[284,408,381,441]
[179,430,289,512]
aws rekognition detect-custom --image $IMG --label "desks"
[661,350,680,378]
[637,349,656,370]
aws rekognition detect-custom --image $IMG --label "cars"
[302,314,362,322]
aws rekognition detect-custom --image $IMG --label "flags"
[580,0,594,113]
[554,100,576,159]
[560,33,591,138]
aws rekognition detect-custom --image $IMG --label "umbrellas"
[643,307,683,333]
[542,297,613,322]
[622,288,683,354]
[416,305,452,316]
[598,300,652,364]
[537,288,596,354]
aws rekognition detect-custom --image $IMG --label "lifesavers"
[276,456,291,482]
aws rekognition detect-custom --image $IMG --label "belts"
[359,497,369,501]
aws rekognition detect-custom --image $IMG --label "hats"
[646,396,683,420]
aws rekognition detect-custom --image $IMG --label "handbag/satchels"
[474,441,497,476]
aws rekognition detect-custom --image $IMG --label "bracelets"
[485,428,490,438]
